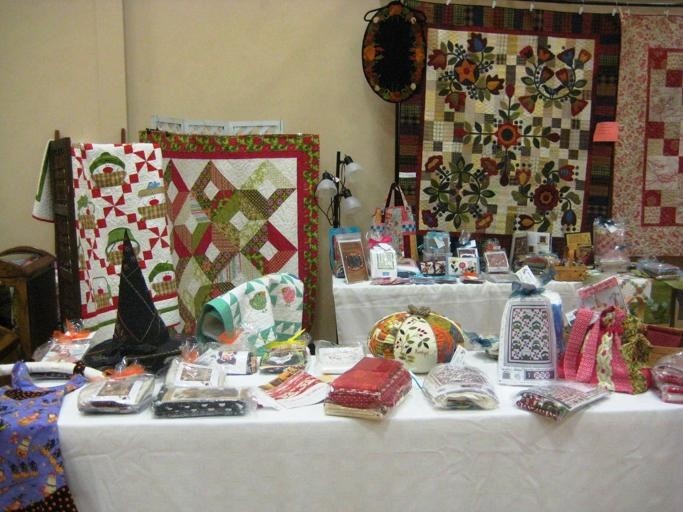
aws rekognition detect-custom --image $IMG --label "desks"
[0,344,683,511]
[327,265,652,344]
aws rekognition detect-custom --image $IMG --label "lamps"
[315,149,364,229]
